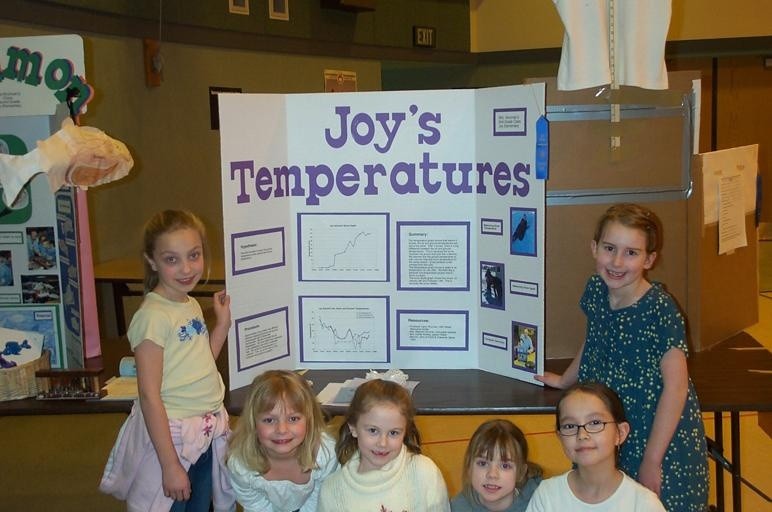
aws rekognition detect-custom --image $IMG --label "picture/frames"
[229,1,250,15]
[269,0,290,22]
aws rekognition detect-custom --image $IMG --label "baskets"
[0,350,54,402]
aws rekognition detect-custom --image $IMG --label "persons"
[316,377,453,512]
[484,268,492,292]
[520,328,535,355]
[220,368,348,512]
[97,207,232,511]
[513,339,530,362]
[530,201,710,511]
[520,379,669,512]
[26,230,57,272]
[445,418,544,512]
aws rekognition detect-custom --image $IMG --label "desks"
[0,326,772,512]
[90,256,232,338]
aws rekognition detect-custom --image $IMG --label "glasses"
[558,418,618,436]
